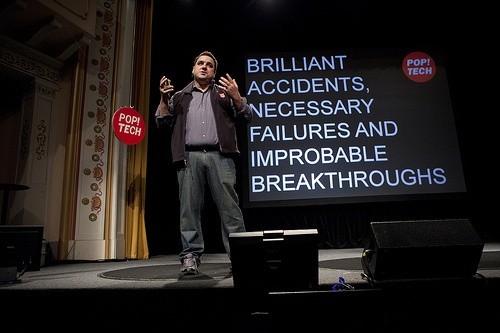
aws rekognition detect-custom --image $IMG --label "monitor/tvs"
[229,228,319,292]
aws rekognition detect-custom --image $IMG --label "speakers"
[0,224,45,272]
[361,218,485,289]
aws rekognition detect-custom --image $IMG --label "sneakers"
[179,254,199,272]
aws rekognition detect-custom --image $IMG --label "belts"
[185,144,220,152]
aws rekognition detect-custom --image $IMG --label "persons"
[155,51,252,279]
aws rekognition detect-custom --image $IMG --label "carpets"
[319,256,362,270]
[99,262,231,280]
[478,251,500,268]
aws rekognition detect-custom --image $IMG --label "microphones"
[211,80,216,84]
